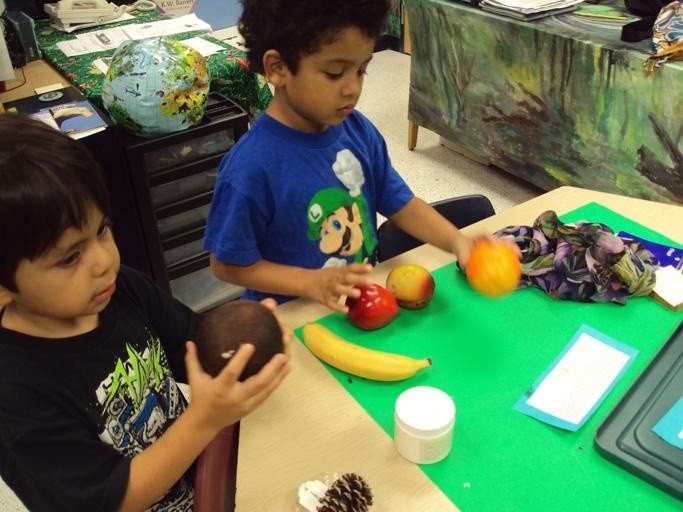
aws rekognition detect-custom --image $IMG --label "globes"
[101,39,212,140]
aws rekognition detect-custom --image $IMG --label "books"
[476,1,584,23]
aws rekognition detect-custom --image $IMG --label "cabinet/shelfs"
[86,79,248,297]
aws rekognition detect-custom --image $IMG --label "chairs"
[377,193,496,263]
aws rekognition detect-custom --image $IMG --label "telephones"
[43,0,117,28]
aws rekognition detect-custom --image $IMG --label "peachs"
[387,263,434,310]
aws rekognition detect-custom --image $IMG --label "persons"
[200,0,525,315]
[0,110,294,512]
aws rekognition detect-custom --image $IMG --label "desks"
[227,183,683,512]
[403,0,683,207]
[26,0,275,130]
[0,56,151,279]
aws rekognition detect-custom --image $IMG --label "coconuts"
[189,298,285,382]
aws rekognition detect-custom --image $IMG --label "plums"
[345,283,399,330]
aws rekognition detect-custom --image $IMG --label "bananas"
[303,323,431,383]
